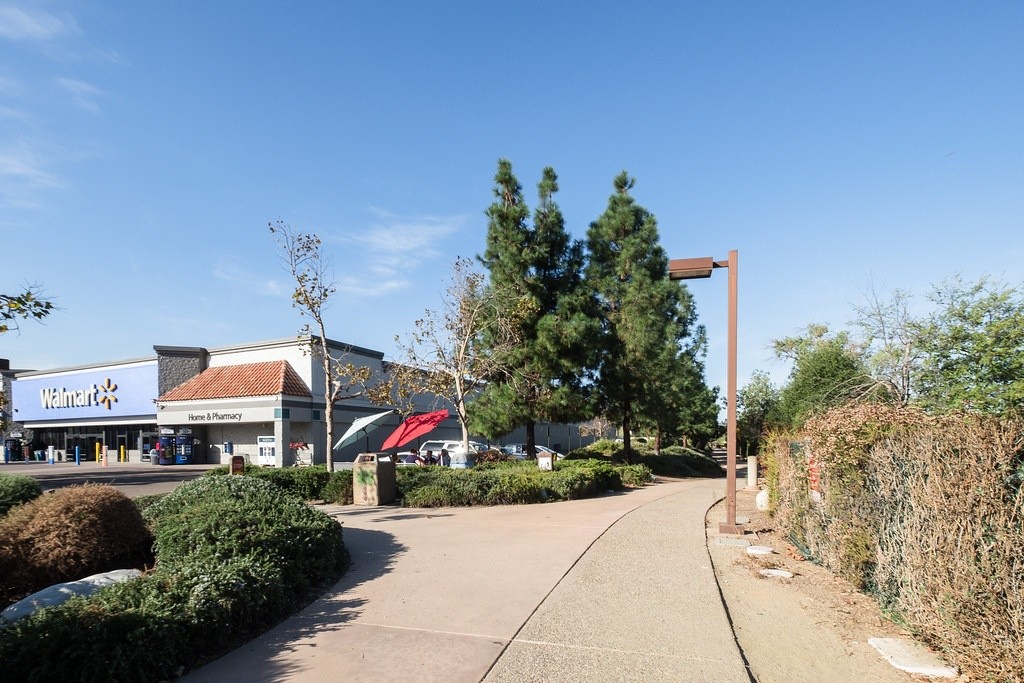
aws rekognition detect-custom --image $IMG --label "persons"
[389,452,402,463]
[415,450,425,461]
[364,450,374,461]
[425,450,434,461]
[406,449,424,464]
[435,449,451,467]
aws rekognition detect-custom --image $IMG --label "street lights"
[668,248,744,534]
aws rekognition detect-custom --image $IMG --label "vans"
[417,439,503,464]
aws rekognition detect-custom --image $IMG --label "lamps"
[13,408,18,412]
[152,399,158,404]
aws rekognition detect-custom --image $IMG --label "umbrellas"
[381,410,449,454]
[333,411,394,452]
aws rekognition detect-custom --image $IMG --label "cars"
[377,451,429,467]
[485,443,566,461]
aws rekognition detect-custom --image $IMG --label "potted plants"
[150,449,160,465]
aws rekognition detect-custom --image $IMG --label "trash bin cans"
[353,453,396,506]
[150,449,159,465]
